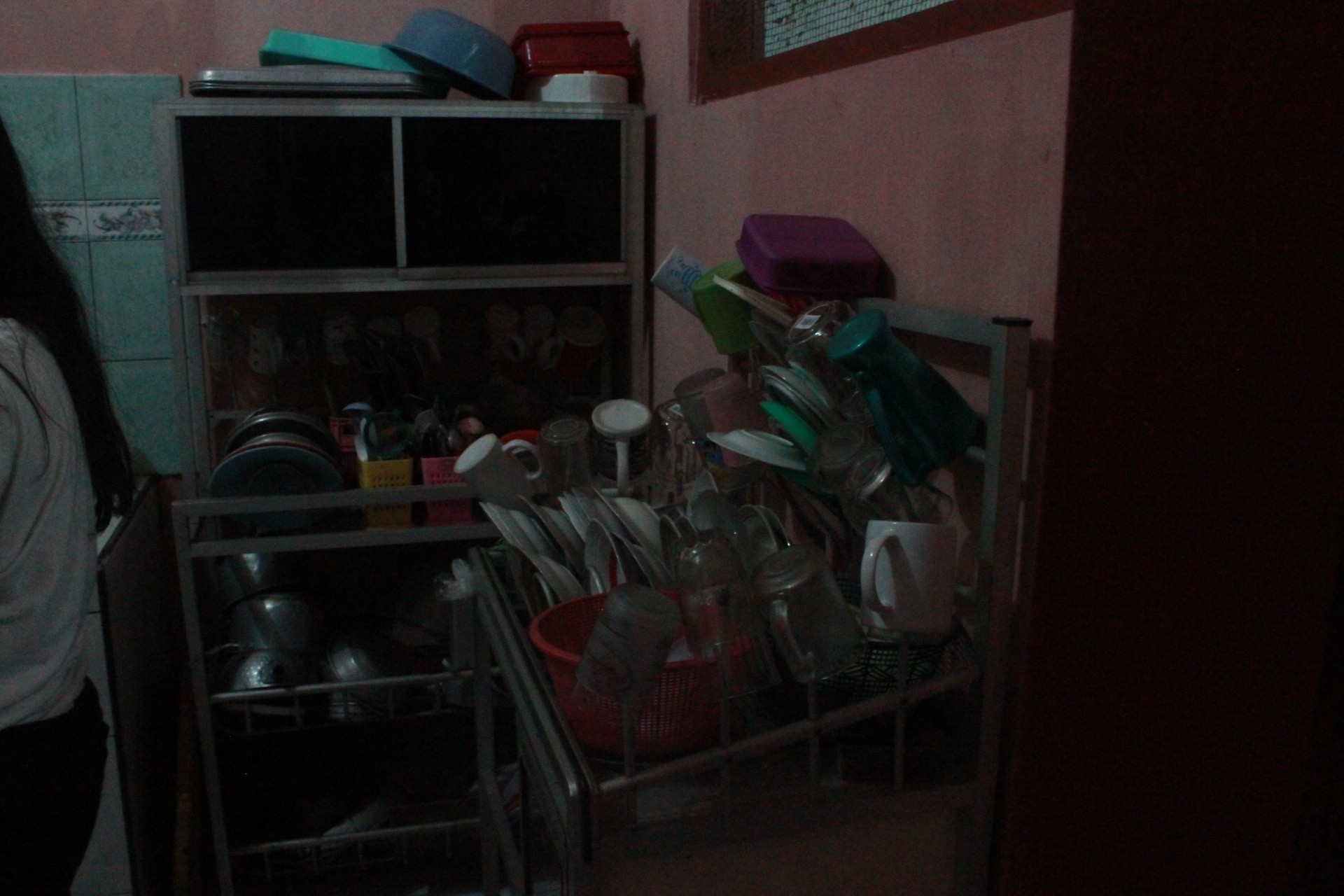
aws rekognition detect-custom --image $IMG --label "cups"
[575,297,986,709]
[455,362,762,508]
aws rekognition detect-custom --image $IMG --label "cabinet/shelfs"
[170,468,486,896]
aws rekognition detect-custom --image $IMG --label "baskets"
[523,587,751,754]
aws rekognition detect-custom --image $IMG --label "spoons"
[343,402,483,460]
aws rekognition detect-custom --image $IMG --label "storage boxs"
[258,33,443,79]
[510,20,638,86]
[736,212,883,294]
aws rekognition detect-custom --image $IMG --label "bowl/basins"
[207,405,399,857]
[389,5,514,100]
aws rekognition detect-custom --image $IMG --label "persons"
[2,118,136,892]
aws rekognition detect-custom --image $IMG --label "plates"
[483,363,816,604]
